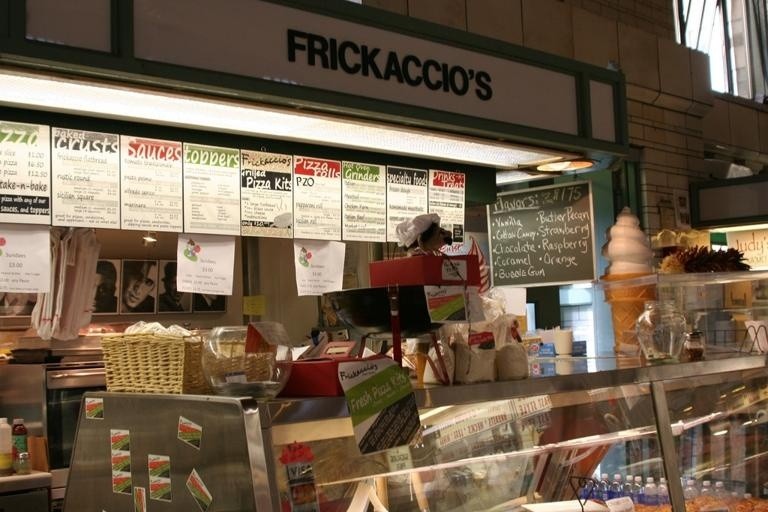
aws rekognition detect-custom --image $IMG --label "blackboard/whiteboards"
[486,179,598,290]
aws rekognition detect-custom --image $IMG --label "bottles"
[634,298,688,363]
[685,331,705,361]
[580,472,753,507]
[0,416,28,472]
[14,451,31,475]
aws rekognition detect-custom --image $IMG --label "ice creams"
[600,205,658,354]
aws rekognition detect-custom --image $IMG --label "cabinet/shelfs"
[531,352,765,511]
[64,348,678,512]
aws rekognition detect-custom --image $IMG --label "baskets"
[206,340,276,383]
[100,333,210,395]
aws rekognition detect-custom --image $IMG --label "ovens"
[0,361,108,512]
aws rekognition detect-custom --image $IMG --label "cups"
[201,320,293,404]
[555,355,574,375]
[553,329,574,355]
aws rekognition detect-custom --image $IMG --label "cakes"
[651,229,708,247]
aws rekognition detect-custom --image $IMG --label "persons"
[192,293,226,311]
[122,261,157,314]
[1,292,35,316]
[395,213,452,356]
[158,262,185,311]
[89,261,118,313]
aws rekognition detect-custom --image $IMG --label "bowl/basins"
[323,285,451,342]
